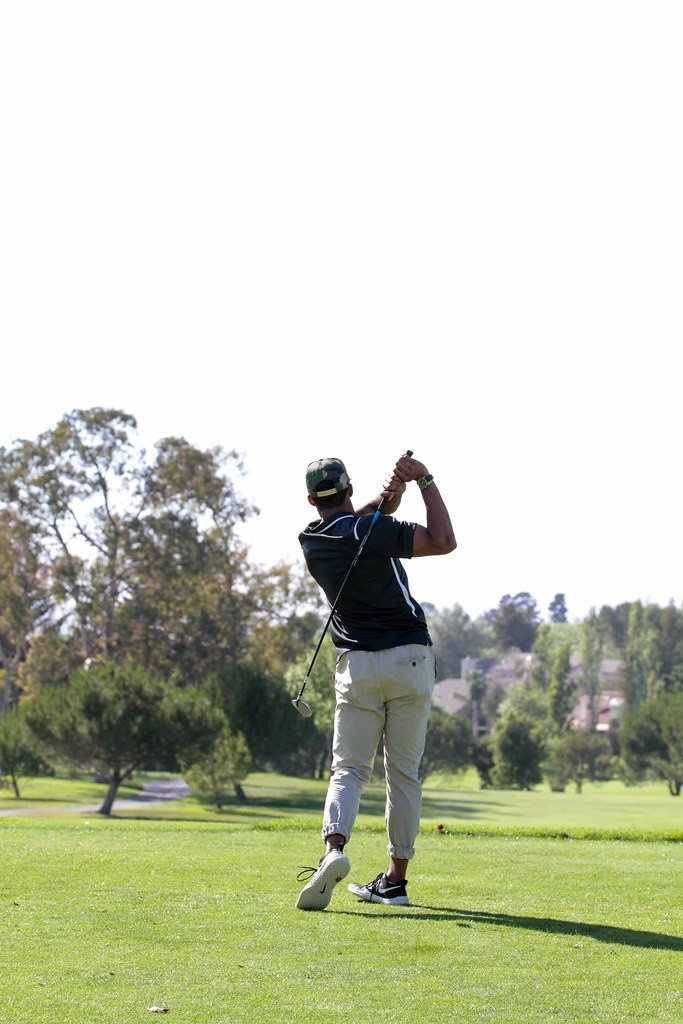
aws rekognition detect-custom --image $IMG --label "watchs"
[417,473,435,490]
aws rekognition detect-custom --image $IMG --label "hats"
[305,457,351,496]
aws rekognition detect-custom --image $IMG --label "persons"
[293,452,460,912]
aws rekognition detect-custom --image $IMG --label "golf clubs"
[291,451,416,716]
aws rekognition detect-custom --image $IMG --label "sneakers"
[347,872,409,906]
[294,844,350,912]
